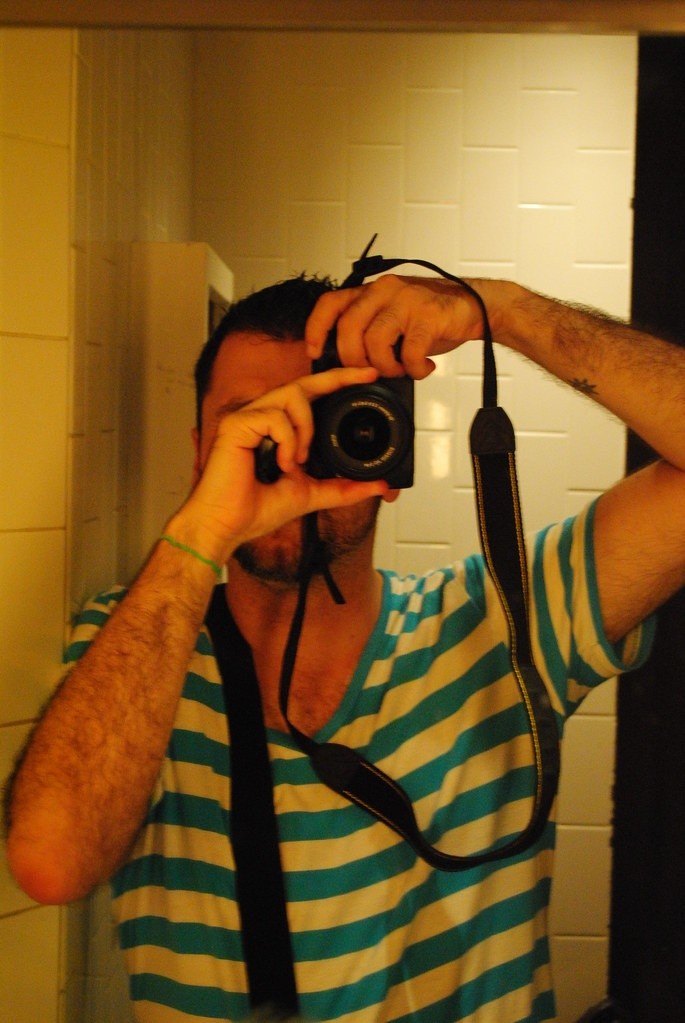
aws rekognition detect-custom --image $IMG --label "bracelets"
[159,533,223,576]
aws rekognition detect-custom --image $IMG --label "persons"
[3,271,685,1022]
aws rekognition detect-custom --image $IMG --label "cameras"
[300,326,414,491]
